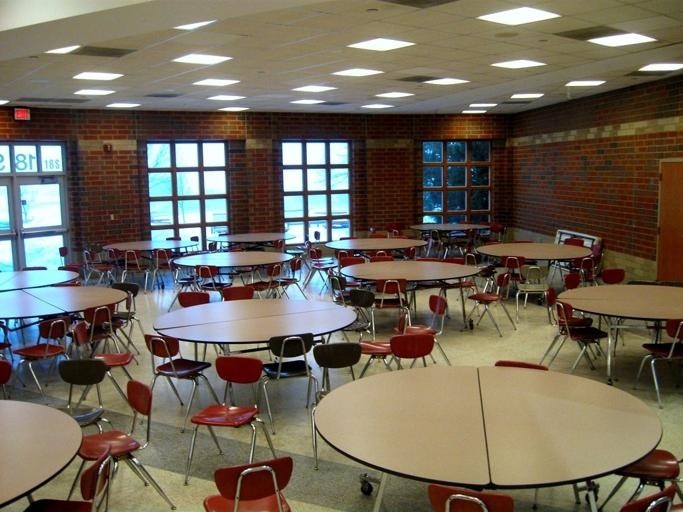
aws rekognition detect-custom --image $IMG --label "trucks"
[210,213,227,235]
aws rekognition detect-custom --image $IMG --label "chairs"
[0,216,682,511]
